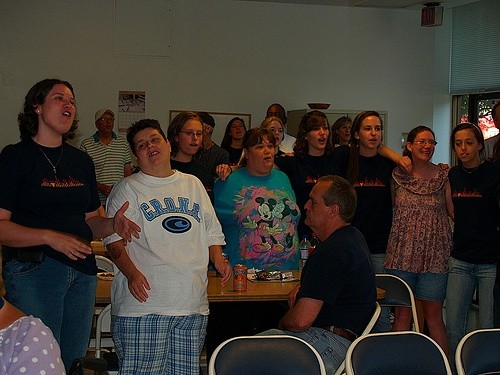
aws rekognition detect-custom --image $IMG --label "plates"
[97,272,114,280]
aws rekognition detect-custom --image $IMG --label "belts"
[327,326,354,341]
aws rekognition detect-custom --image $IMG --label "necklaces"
[32,142,65,174]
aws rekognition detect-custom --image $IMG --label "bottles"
[300,234,311,271]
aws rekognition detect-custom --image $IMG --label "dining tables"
[91,270,384,359]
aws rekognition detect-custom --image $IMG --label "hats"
[200,113,215,128]
[95,107,115,121]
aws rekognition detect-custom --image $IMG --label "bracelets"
[228,165,233,174]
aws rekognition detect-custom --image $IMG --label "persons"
[331,116,356,149]
[215,111,412,246]
[257,176,378,375]
[383,126,449,363]
[0,78,142,375]
[265,104,297,153]
[217,111,247,165]
[81,108,131,211]
[104,119,231,375]
[450,123,500,375]
[489,104,500,163]
[0,273,66,375]
[281,111,451,333]
[257,116,298,164]
[207,128,302,375]
[198,112,230,178]
[167,112,213,210]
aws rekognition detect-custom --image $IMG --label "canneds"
[216,252,228,277]
[234,264,247,292]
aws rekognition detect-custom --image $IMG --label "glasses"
[102,117,114,122]
[414,140,437,146]
[179,130,202,135]
[267,112,280,116]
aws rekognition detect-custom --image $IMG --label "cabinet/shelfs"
[287,108,388,149]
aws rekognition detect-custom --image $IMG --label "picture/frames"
[169,109,252,150]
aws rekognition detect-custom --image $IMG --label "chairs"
[209,274,500,375]
